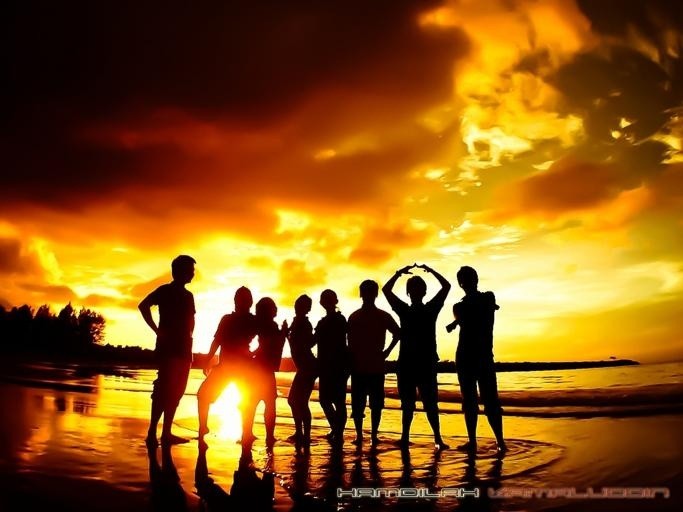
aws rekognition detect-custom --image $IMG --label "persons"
[136,254,195,445]
[381,263,452,450]
[314,290,350,443]
[284,295,321,440]
[196,286,265,442]
[451,267,509,453]
[253,296,287,444]
[346,280,400,444]
